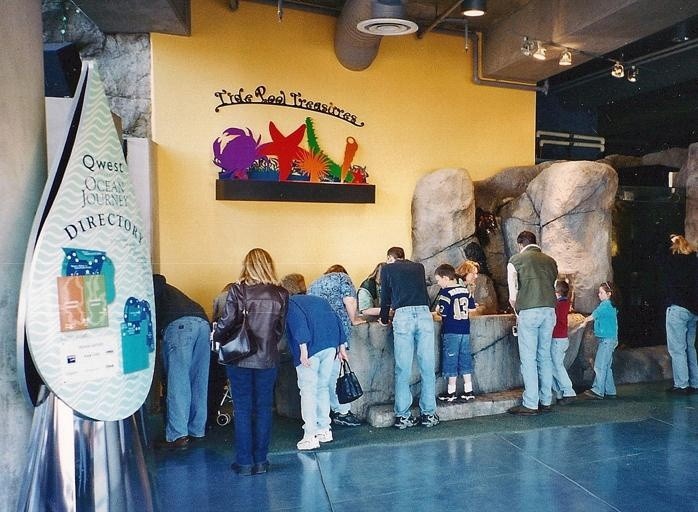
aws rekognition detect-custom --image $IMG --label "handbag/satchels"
[336,373,363,404]
[219,317,258,365]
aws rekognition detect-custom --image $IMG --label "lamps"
[461,0,488,17]
[520,35,639,82]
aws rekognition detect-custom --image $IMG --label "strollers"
[207,332,236,426]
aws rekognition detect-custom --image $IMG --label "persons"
[208,248,289,475]
[280,274,347,450]
[358,263,394,322]
[456,260,484,315]
[378,246,440,429]
[307,265,366,428]
[581,281,622,399]
[154,273,213,450]
[506,231,558,417]
[655,234,698,395]
[551,281,578,406]
[435,264,477,403]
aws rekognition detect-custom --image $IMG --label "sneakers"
[231,461,269,475]
[153,436,190,451]
[296,411,362,450]
[394,391,476,430]
[666,386,697,395]
[509,388,616,416]
